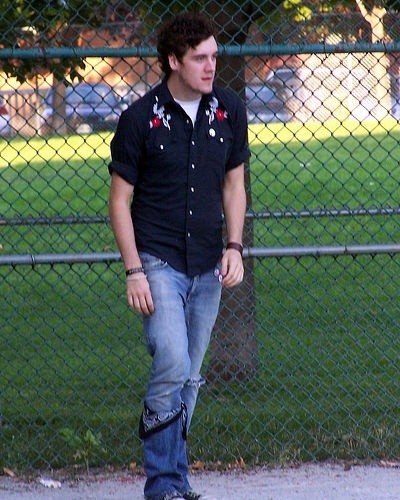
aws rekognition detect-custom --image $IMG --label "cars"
[0,93,11,140]
[37,83,131,136]
[269,66,299,91]
[246,81,293,124]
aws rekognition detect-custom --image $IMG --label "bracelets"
[126,267,144,276]
[226,242,244,256]
[126,274,148,280]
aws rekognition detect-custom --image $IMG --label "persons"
[105,13,250,499]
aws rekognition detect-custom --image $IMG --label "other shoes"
[148,490,201,500]
